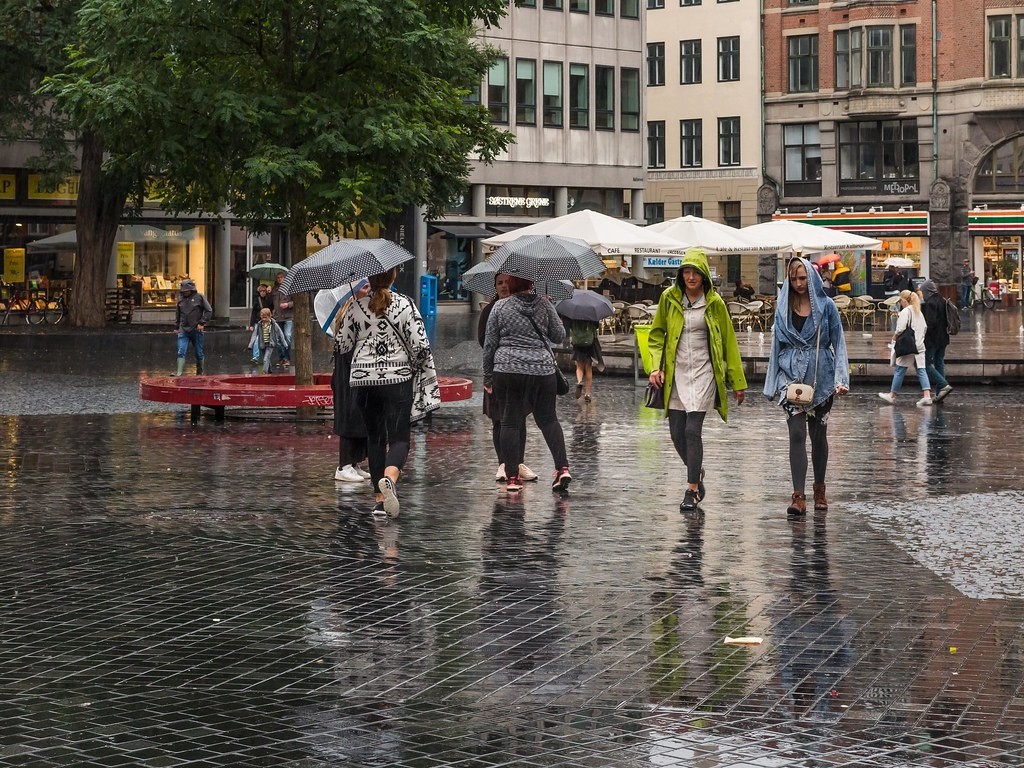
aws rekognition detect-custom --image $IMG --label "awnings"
[432,224,520,237]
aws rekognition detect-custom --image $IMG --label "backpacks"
[946,297,961,335]
[572,319,594,346]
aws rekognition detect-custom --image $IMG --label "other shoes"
[284,360,291,366]
[276,360,283,365]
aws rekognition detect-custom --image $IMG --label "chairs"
[597,293,904,335]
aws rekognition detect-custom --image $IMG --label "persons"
[483,276,571,490]
[883,265,912,313]
[813,262,852,298]
[331,268,441,518]
[879,280,952,404]
[647,249,748,509]
[960,259,975,308]
[618,260,630,273]
[763,256,849,513]
[477,274,538,481]
[566,317,599,400]
[248,273,294,374]
[171,279,212,376]
[733,280,755,302]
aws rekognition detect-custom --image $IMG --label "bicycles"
[2,280,47,325]
[968,283,995,309]
[44,285,72,325]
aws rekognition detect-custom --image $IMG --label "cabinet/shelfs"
[142,276,184,307]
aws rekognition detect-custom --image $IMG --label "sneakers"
[379,476,400,519]
[680,489,700,510]
[372,501,386,515]
[933,384,953,403]
[698,467,705,502]
[879,392,896,404]
[576,383,583,399]
[507,476,523,491]
[496,462,507,481]
[519,464,538,480]
[354,465,371,479]
[585,393,592,403]
[915,397,932,407]
[335,464,364,482]
[552,467,572,491]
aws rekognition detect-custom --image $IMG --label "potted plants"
[996,256,1018,307]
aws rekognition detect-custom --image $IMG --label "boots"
[196,359,205,375]
[170,359,187,377]
[787,493,806,514]
[813,483,829,510]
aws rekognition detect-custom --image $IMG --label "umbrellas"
[554,289,614,322]
[27,230,76,253]
[486,234,608,296]
[278,237,415,337]
[116,225,270,246]
[481,209,883,281]
[249,263,290,281]
[460,262,575,300]
[884,258,912,267]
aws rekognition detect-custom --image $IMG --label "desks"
[867,298,886,326]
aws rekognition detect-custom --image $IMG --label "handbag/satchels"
[644,377,664,409]
[786,384,814,405]
[555,362,569,395]
[894,327,920,358]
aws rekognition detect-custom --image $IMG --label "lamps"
[840,206,854,214]
[974,203,988,213]
[899,205,913,213]
[869,206,883,213]
[807,206,820,218]
[775,208,788,216]
